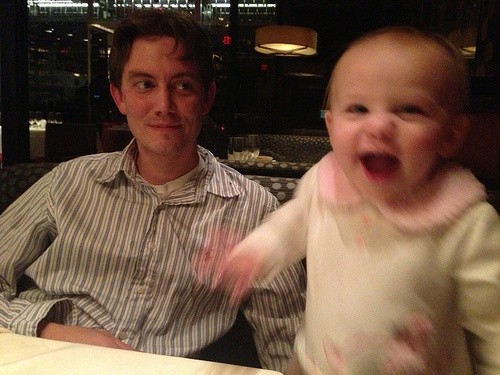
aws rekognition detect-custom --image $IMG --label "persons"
[203,27,499,374]
[0,9,305,373]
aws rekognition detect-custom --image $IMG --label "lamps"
[254,23,317,59]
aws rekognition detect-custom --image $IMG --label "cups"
[227,134,260,160]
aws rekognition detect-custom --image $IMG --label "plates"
[257,156,273,161]
